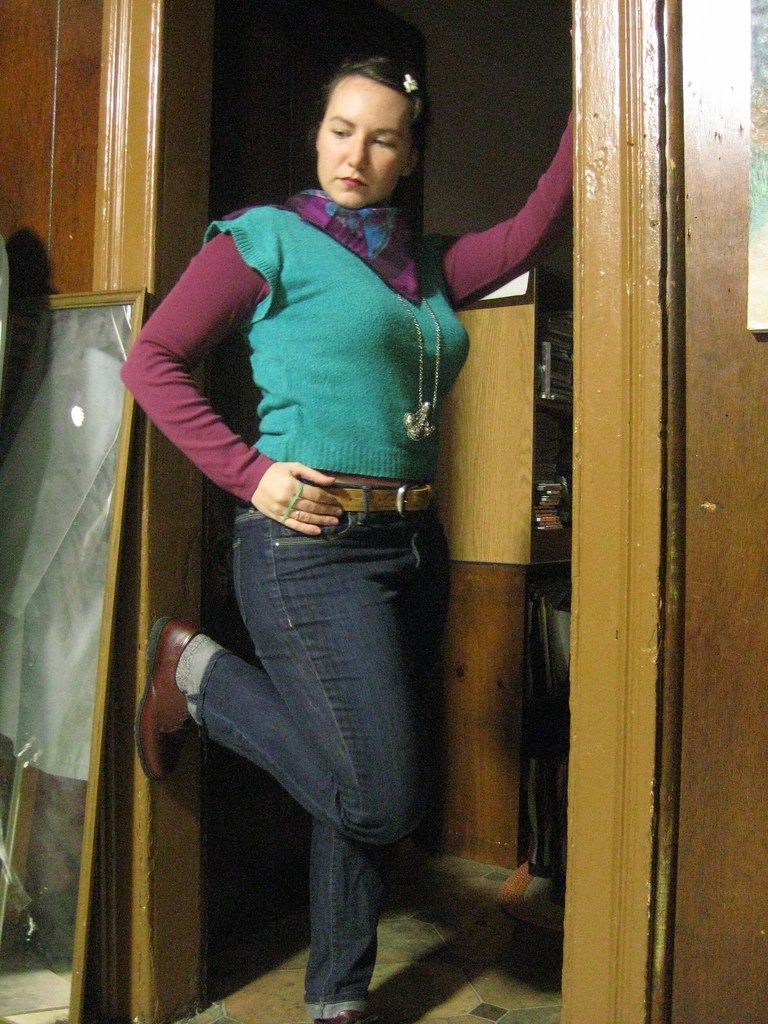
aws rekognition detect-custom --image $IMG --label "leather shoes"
[314,1010,379,1024]
[133,614,205,781]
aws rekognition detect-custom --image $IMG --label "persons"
[121,54,574,1024]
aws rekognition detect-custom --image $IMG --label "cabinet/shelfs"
[435,264,573,869]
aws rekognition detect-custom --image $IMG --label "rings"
[295,510,300,520]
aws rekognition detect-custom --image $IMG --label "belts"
[321,483,432,516]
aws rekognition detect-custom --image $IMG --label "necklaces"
[398,293,440,442]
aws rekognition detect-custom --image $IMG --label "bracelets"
[280,483,304,525]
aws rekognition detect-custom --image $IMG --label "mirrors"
[0,286,150,1024]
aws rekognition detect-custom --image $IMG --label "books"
[534,473,569,531]
[538,328,573,403]
[524,573,570,700]
